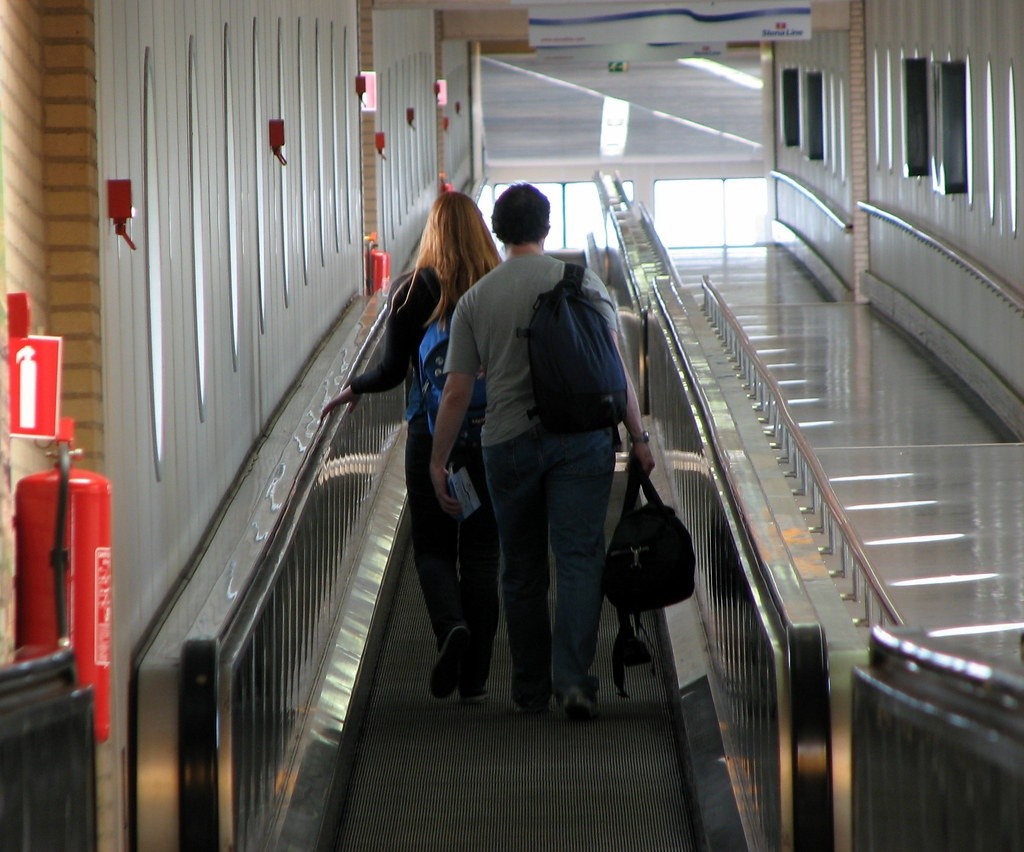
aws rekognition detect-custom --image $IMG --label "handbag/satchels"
[602,455,695,698]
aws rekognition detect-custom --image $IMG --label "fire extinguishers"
[369,250,389,296]
[13,417,111,737]
[441,177,453,191]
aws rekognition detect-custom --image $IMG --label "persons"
[319,190,507,708]
[427,182,657,721]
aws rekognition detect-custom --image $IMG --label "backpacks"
[516,261,629,437]
[404,265,487,441]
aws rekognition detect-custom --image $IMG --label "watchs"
[629,430,650,443]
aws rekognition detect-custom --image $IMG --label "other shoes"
[562,685,593,715]
[516,693,550,714]
[429,622,470,698]
[457,678,489,702]
